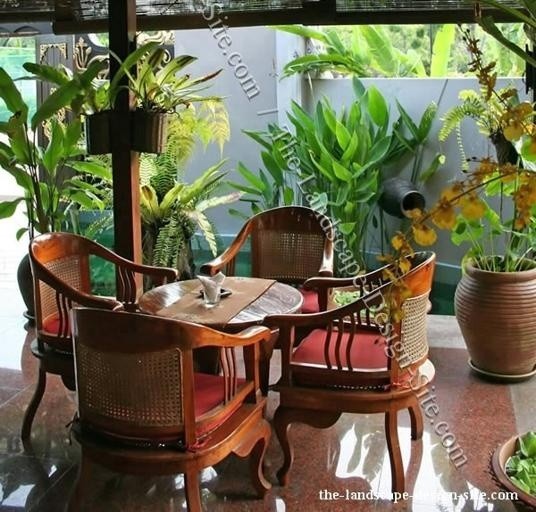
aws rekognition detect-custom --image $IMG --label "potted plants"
[440,84,536,381]
[106,43,223,154]
[74,61,131,153]
[488,431,536,511]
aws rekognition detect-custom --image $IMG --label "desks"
[139,277,304,468]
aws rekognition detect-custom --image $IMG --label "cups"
[203,286,221,308]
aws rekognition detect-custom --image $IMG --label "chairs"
[21,232,179,441]
[66,308,273,511]
[200,205,334,417]
[265,251,437,500]
